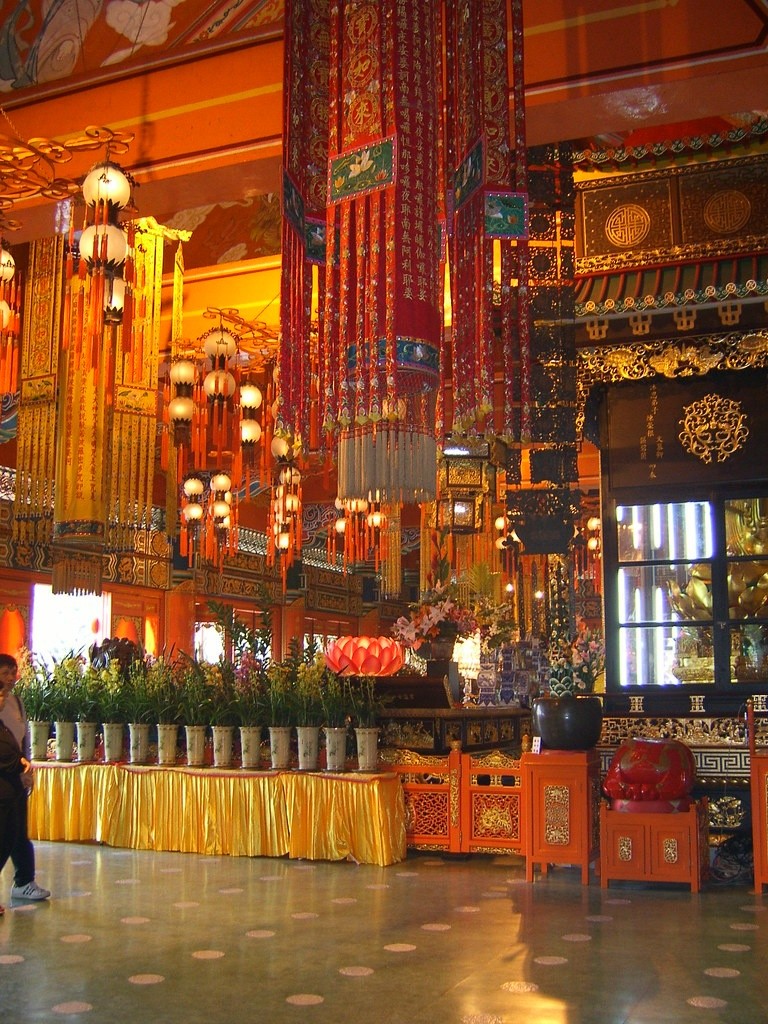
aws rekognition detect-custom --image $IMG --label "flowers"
[480,603,514,663]
[11,646,383,729]
[566,630,606,694]
[392,523,479,650]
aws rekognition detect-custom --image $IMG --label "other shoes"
[0,905,5,915]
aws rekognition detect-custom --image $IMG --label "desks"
[10,756,409,867]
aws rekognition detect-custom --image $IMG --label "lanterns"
[237,384,264,411]
[496,513,522,551]
[169,361,198,389]
[103,270,127,326]
[269,428,303,552]
[169,397,194,430]
[237,418,261,447]
[324,635,405,677]
[181,469,231,550]
[1,299,11,331]
[0,248,16,286]
[572,515,603,563]
[79,219,128,271]
[204,371,237,401]
[81,160,130,210]
[332,495,387,538]
[204,331,238,363]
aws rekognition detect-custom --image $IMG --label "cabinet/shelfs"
[750,749,768,893]
[520,750,602,885]
[599,797,708,893]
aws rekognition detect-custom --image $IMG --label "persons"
[0,681,35,914]
[0,652,52,900]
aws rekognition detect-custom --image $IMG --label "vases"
[102,723,124,762]
[156,724,179,765]
[324,728,347,771]
[499,636,551,706]
[54,722,75,762]
[211,726,235,766]
[75,722,97,761]
[268,727,292,769]
[29,721,50,760]
[240,727,262,767]
[425,637,455,661]
[354,728,378,770]
[296,727,320,770]
[128,724,151,762]
[184,725,206,766]
[529,696,603,752]
[477,664,496,706]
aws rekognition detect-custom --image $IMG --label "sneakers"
[10,879,51,900]
